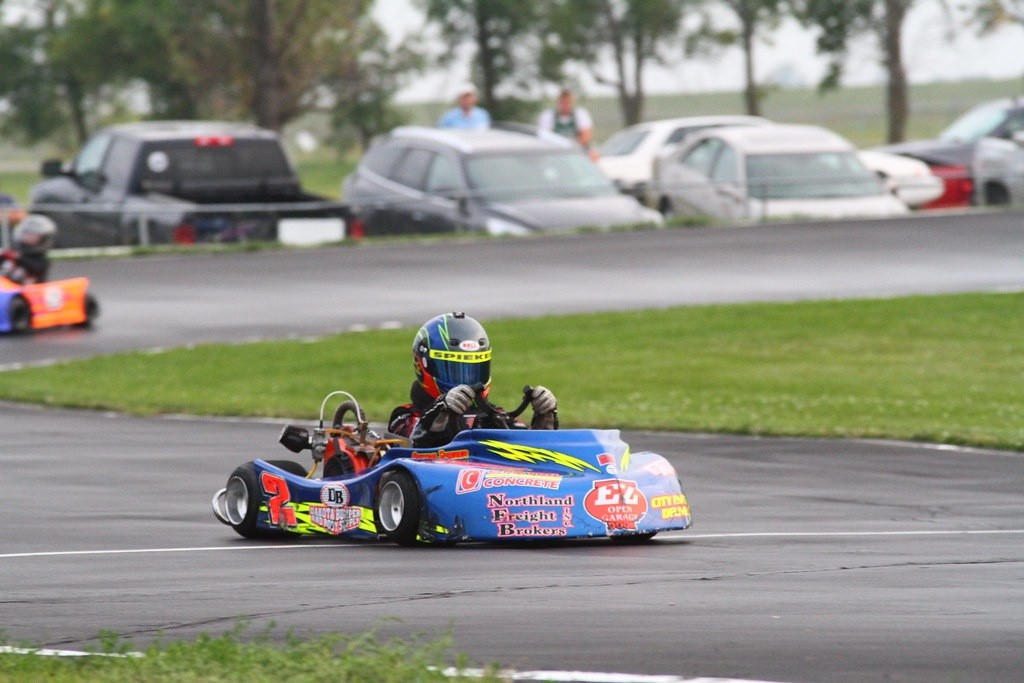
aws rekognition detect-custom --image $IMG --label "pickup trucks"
[30,121,369,246]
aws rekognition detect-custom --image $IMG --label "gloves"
[531,385,557,415]
[445,384,476,415]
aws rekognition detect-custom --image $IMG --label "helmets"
[413,311,492,399]
[13,215,56,255]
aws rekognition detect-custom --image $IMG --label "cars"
[590,114,776,196]
[651,123,909,224]
[859,94,1024,224]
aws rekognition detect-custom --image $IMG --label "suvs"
[338,119,665,237]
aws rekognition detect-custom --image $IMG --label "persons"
[389,313,559,450]
[540,87,599,163]
[439,82,490,130]
[0,215,57,284]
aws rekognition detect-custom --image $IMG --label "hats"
[456,84,476,98]
